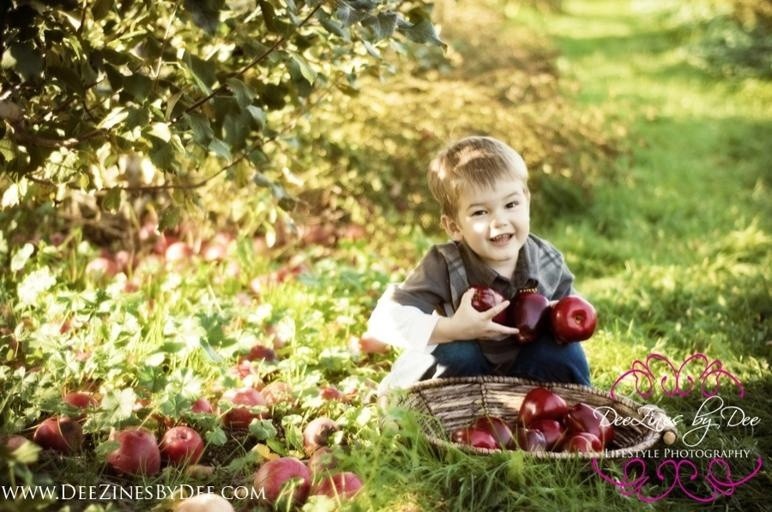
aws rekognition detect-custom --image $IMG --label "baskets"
[383,377,678,480]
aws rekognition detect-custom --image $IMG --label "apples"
[0,217,615,512]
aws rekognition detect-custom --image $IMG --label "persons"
[365,132,590,392]
